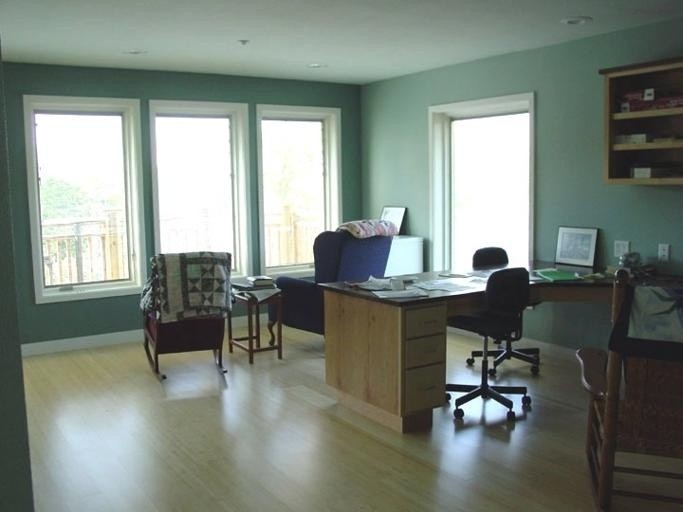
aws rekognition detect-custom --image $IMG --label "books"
[246,275,274,286]
[537,269,584,282]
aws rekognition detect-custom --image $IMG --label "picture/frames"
[380,206,406,236]
[554,225,599,269]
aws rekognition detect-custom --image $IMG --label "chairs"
[575,264,683,512]
[267,220,398,346]
[467,247,540,376]
[445,268,531,420]
[139,251,232,381]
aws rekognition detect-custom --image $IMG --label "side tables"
[228,290,284,364]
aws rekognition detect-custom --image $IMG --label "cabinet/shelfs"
[598,56,683,186]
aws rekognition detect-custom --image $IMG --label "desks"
[317,260,615,436]
[384,235,424,277]
